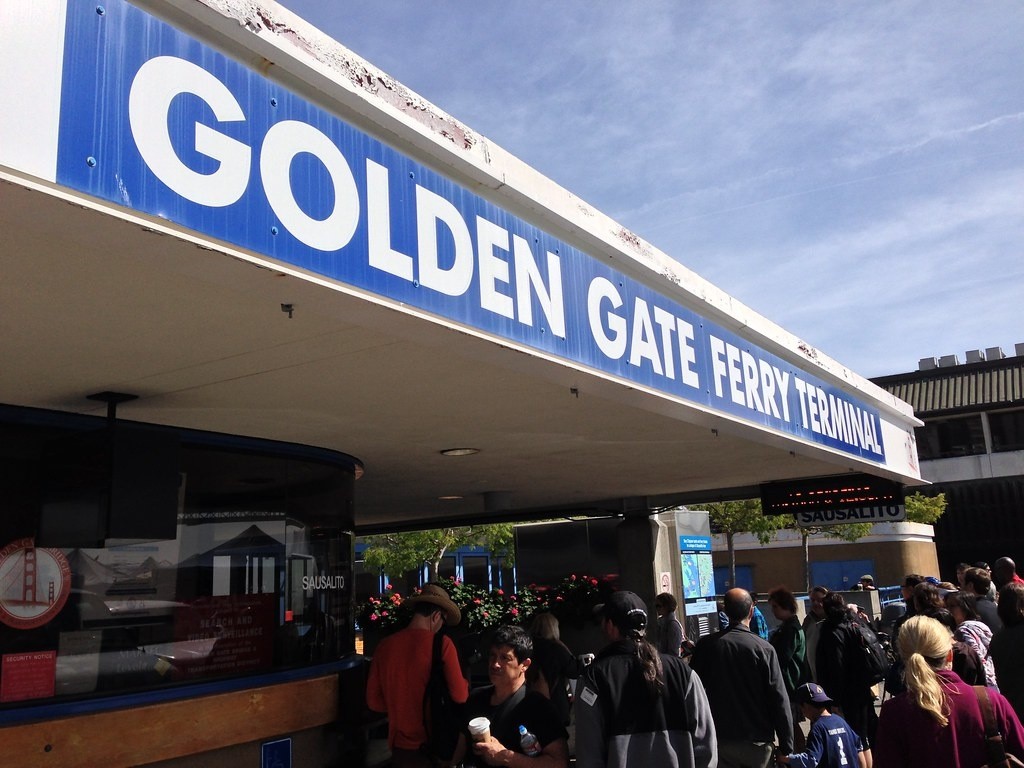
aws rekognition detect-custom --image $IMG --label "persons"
[362,556,1024,768]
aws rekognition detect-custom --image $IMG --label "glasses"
[655,604,663,609]
[441,614,449,628]
[900,586,907,589]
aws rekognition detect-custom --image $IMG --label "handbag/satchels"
[671,619,695,664]
[970,684,1024,768]
[421,632,461,762]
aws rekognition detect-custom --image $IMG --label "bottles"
[519,726,542,757]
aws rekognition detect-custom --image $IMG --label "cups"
[858,584,863,591]
[468,717,491,743]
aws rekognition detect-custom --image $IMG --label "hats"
[405,584,462,626]
[926,576,943,587]
[860,575,874,581]
[937,588,959,599]
[796,682,833,702]
[594,591,648,635]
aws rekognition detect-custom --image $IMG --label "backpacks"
[835,621,888,684]
